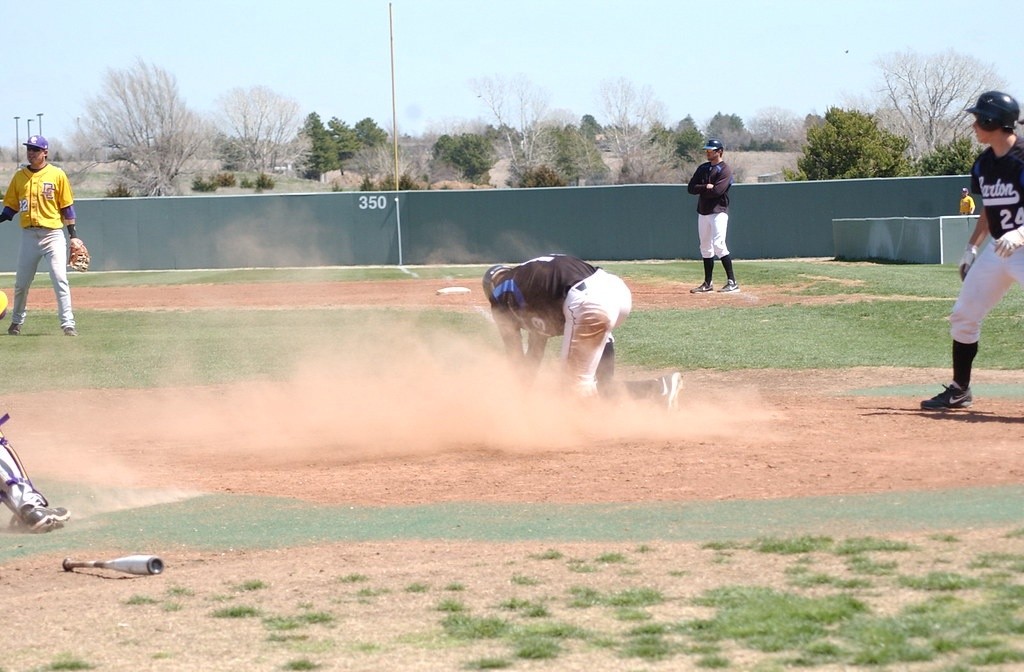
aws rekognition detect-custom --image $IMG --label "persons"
[0,135,91,338]
[0,436,72,533]
[483,254,682,416]
[960,188,975,215]
[920,92,1024,410]
[688,140,739,293]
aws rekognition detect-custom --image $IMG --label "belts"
[575,282,586,291]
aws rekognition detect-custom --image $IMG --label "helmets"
[482,265,512,302]
[964,90,1019,130]
[702,140,724,150]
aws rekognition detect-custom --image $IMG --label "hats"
[23,135,48,149]
[961,187,968,192]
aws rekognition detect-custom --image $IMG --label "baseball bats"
[62,554,164,575]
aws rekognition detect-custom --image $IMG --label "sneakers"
[24,508,71,533]
[717,279,739,292]
[658,372,683,414]
[64,328,77,337]
[690,281,714,294]
[8,323,21,335]
[920,384,972,409]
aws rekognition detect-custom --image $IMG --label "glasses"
[705,150,716,152]
[27,146,40,152]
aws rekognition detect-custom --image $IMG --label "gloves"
[993,226,1024,258]
[959,243,977,282]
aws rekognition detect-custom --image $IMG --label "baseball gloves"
[66,238,91,273]
